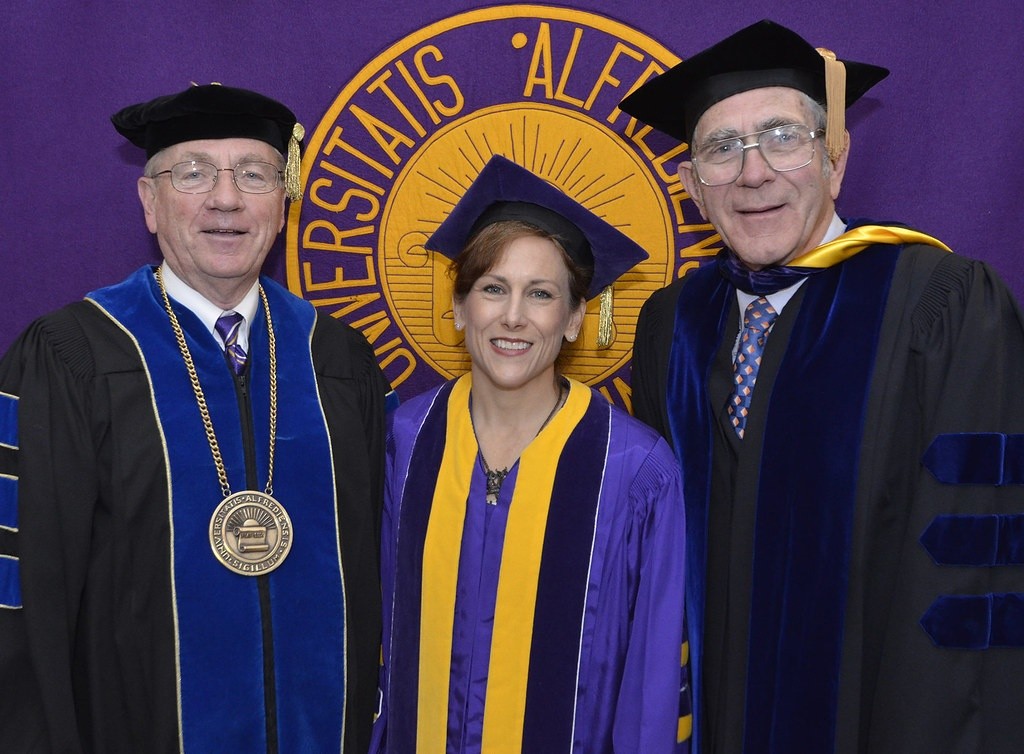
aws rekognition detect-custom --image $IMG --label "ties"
[215,312,248,376]
[725,298,778,439]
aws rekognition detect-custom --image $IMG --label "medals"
[208,490,293,576]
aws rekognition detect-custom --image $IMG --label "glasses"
[692,124,826,186]
[150,159,283,195]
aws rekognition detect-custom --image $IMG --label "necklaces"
[468,380,565,507]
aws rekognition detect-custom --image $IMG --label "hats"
[425,154,649,348]
[109,81,306,202]
[617,19,891,163]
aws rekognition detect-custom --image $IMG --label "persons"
[369,155,689,754]
[0,86,386,754]
[615,16,1024,754]
